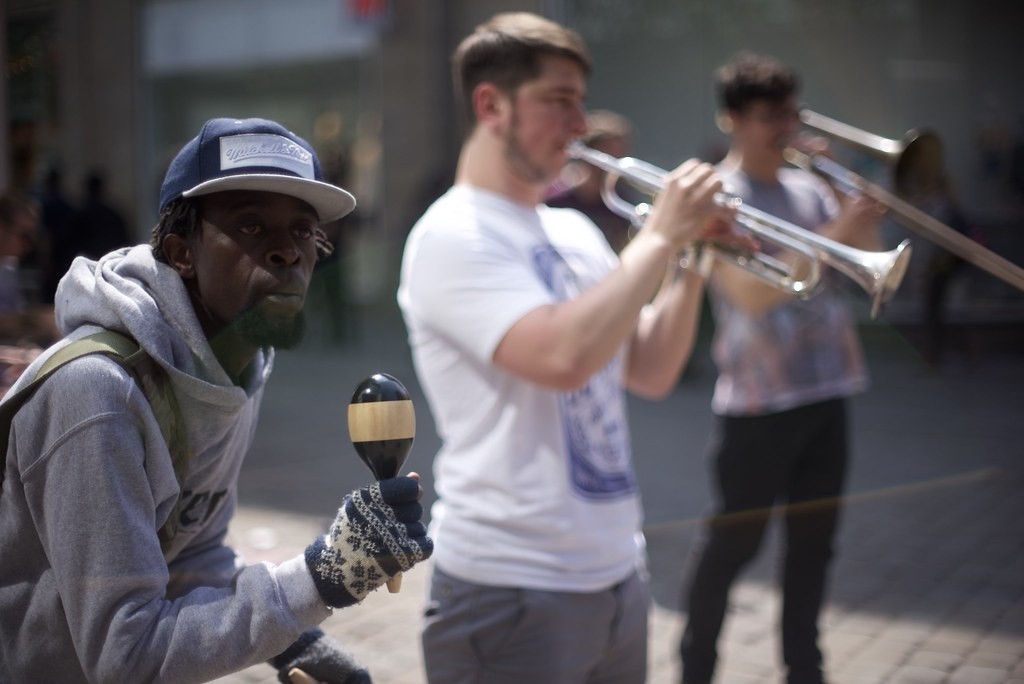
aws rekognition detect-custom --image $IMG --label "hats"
[160,118,355,221]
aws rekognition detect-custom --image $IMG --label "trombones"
[787,106,1024,289]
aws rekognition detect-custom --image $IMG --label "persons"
[395,9,762,684]
[535,106,645,255]
[677,49,887,684]
[0,116,434,684]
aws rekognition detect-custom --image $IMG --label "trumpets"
[564,140,912,333]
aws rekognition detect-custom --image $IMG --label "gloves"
[304,478,433,609]
[269,628,373,684]
